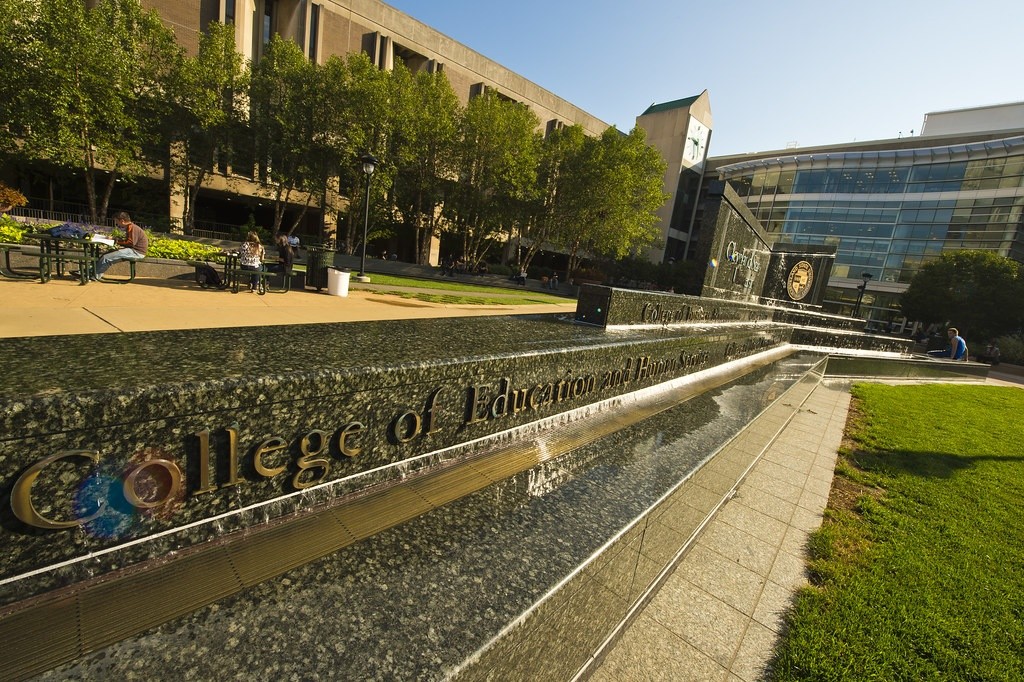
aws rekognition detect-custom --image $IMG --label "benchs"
[0,244,137,286]
[188,260,298,295]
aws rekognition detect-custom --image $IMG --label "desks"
[22,232,102,284]
[213,252,276,295]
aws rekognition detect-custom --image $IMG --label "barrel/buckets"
[302,245,338,288]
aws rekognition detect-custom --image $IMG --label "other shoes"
[85,276,96,281]
[247,283,252,289]
[69,270,85,278]
[251,289,256,293]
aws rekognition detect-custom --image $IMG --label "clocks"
[683,115,710,169]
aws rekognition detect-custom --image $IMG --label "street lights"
[854,272,873,318]
[664,255,676,291]
[351,152,380,283]
[851,285,863,318]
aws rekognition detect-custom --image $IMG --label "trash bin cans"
[305,246,338,292]
[925,333,948,354]
[326,265,352,297]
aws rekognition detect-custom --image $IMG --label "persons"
[265,234,293,292]
[288,233,300,258]
[929,328,968,362]
[440,256,488,280]
[240,231,264,292]
[68,212,148,280]
[517,267,527,286]
